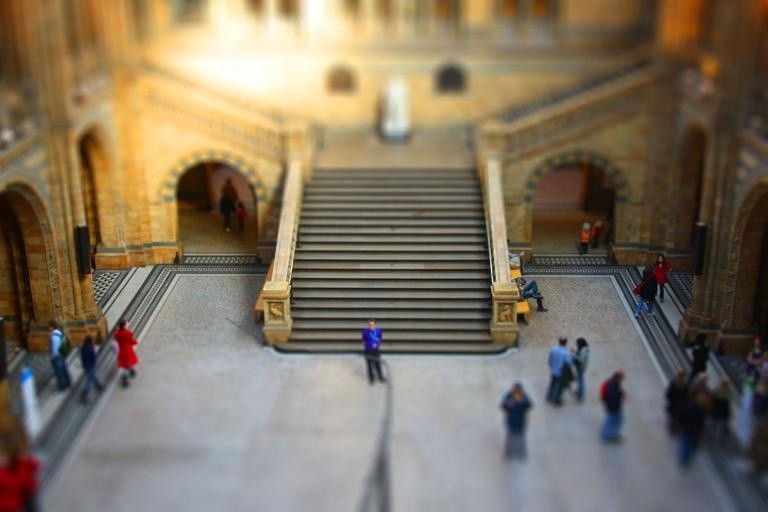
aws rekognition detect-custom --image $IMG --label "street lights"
[560,365,573,385]
[632,283,643,295]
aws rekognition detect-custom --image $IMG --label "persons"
[47,320,70,390]
[219,178,250,232]
[362,320,386,384]
[580,220,603,254]
[546,333,767,474]
[507,239,549,312]
[499,384,532,462]
[632,253,674,318]
[80,335,104,406]
[113,320,141,388]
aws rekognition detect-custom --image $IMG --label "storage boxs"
[510,267,530,326]
[254,259,275,324]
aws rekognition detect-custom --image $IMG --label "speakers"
[81,391,90,406]
[93,378,103,392]
[121,368,135,388]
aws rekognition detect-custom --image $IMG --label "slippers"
[52,331,71,358]
[598,378,608,400]
